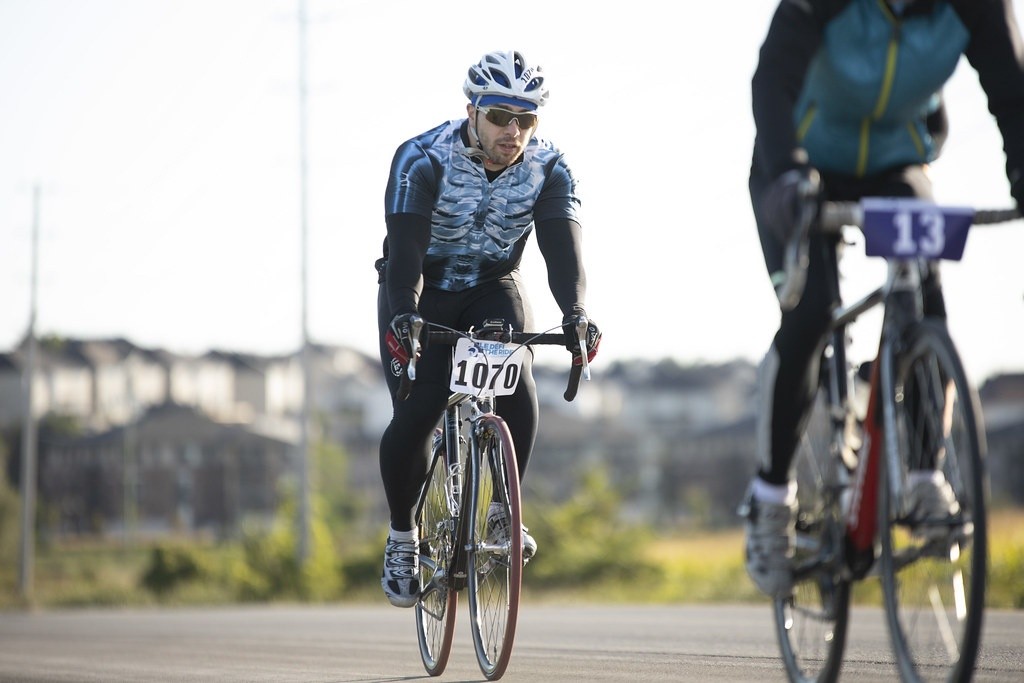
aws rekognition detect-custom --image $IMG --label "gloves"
[385,311,423,364]
[562,308,602,366]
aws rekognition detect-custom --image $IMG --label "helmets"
[463,49,550,106]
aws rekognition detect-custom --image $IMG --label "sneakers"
[486,502,537,560]
[899,470,971,541]
[738,475,802,597]
[382,533,422,608]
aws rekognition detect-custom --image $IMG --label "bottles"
[840,360,880,469]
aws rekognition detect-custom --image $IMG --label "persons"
[379,52,602,609]
[745,0,1024,599]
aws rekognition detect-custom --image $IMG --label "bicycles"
[743,146,1024,681]
[406,311,595,683]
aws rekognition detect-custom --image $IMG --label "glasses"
[476,106,539,131]
